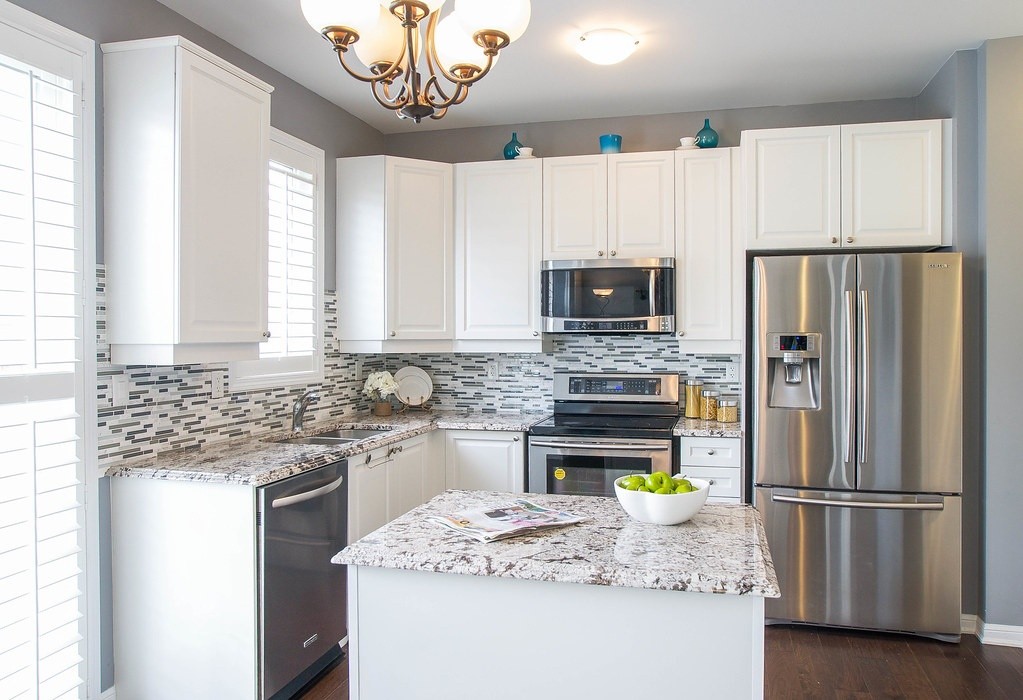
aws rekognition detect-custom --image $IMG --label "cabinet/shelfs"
[337,116,955,355]
[342,428,744,549]
[98,35,274,365]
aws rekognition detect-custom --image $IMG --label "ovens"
[528,436,675,498]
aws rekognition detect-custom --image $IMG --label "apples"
[619,471,699,494]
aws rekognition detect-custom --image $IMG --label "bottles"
[717,401,738,422]
[504,133,524,159]
[696,119,719,148]
[700,390,720,420]
[685,379,704,418]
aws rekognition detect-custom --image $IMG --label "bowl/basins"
[614,474,710,526]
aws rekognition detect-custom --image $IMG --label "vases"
[503,131,523,160]
[374,400,392,417]
[695,119,719,149]
[598,134,622,154]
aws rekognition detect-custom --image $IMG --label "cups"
[600,135,622,154]
[515,146,533,156]
[680,137,700,146]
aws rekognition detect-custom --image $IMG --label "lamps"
[593,287,614,316]
[297,0,530,124]
[579,28,639,65]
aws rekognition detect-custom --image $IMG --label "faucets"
[291,388,334,433]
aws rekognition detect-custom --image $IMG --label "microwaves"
[540,258,676,332]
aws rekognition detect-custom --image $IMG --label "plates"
[514,156,536,159]
[393,366,433,405]
[676,146,700,149]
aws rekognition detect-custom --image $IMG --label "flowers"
[361,370,400,403]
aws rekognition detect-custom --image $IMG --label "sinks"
[271,437,356,446]
[316,423,389,440]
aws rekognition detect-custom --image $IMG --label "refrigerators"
[750,253,966,641]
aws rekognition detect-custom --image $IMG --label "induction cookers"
[529,373,680,438]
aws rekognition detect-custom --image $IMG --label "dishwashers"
[256,458,348,700]
